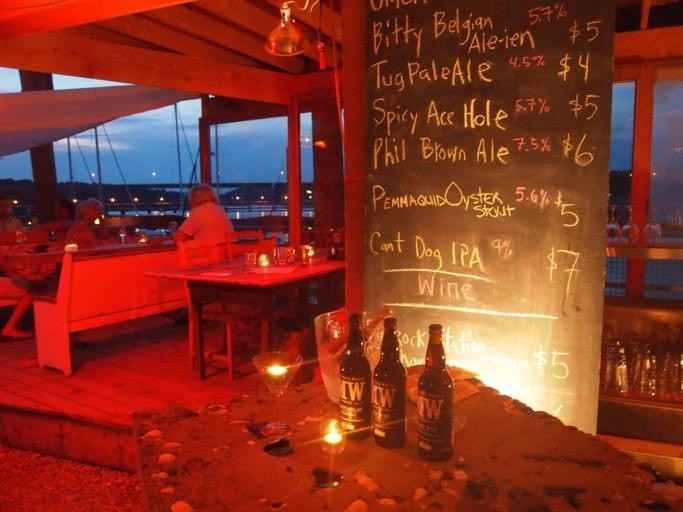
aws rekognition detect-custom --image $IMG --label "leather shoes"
[2,326,32,341]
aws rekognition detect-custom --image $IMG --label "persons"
[172,184,238,247]
[62,198,120,249]
[0,277,34,339]
[0,197,75,232]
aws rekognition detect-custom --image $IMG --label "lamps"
[264,0,329,71]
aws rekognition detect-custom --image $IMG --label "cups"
[245,246,312,269]
[314,308,396,406]
[600,336,683,405]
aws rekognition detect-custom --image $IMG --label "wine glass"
[640,204,665,247]
[606,204,622,245]
[252,351,303,436]
[12,227,57,249]
[620,204,640,247]
[117,222,179,244]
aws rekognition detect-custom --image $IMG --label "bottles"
[339,312,373,440]
[372,317,408,446]
[418,324,453,462]
[334,309,394,365]
[327,241,340,261]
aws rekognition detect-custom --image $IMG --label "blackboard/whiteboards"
[363,0,615,437]
